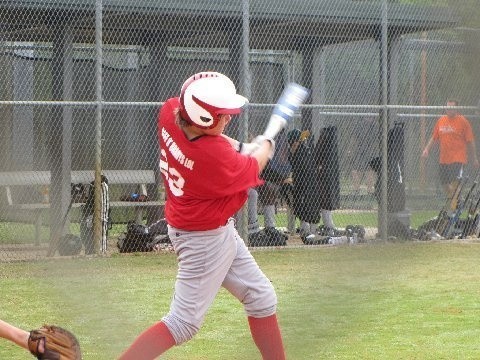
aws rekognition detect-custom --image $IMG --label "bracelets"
[237,141,242,153]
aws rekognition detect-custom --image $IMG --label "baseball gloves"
[26,327,80,360]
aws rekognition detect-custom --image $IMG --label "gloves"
[251,136,275,160]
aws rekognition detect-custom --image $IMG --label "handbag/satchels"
[81,175,110,254]
[117,221,149,253]
[149,219,170,252]
[58,233,81,255]
[249,226,287,246]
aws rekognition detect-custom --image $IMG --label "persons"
[0,318,81,360]
[248,156,295,234]
[286,129,321,233]
[420,100,478,214]
[118,72,286,360]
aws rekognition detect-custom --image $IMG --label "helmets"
[288,128,305,143]
[178,71,249,129]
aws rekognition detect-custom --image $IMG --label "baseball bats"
[442,180,477,238]
[47,187,81,256]
[433,177,463,232]
[437,176,469,235]
[265,83,308,141]
